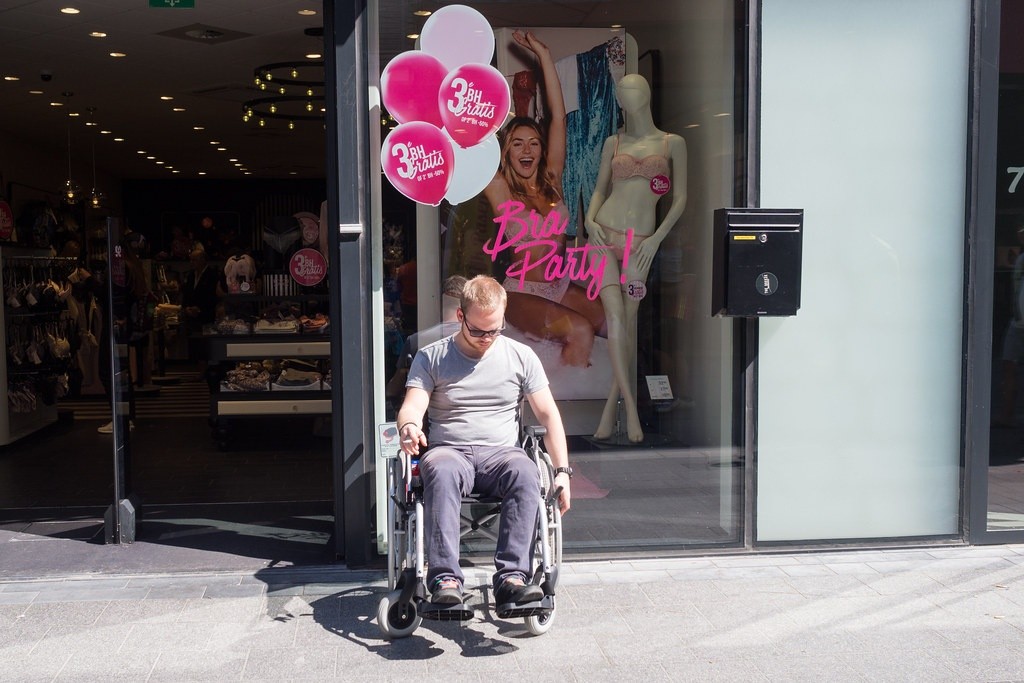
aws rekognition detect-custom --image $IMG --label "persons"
[393,275,467,392]
[396,275,570,605]
[483,32,606,369]
[584,73,687,442]
[181,249,218,382]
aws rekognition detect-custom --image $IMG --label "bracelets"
[398,422,418,436]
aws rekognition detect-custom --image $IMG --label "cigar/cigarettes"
[403,438,420,443]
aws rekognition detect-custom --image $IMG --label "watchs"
[553,466,572,480]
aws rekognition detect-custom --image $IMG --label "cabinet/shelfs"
[207,296,334,416]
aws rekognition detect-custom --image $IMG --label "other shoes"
[98,419,134,434]
[494,577,545,611]
[432,578,464,604]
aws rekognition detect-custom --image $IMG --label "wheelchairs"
[375,421,562,638]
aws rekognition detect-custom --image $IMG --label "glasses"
[462,310,506,337]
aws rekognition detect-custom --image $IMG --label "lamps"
[89,104,102,209]
[65,92,77,204]
